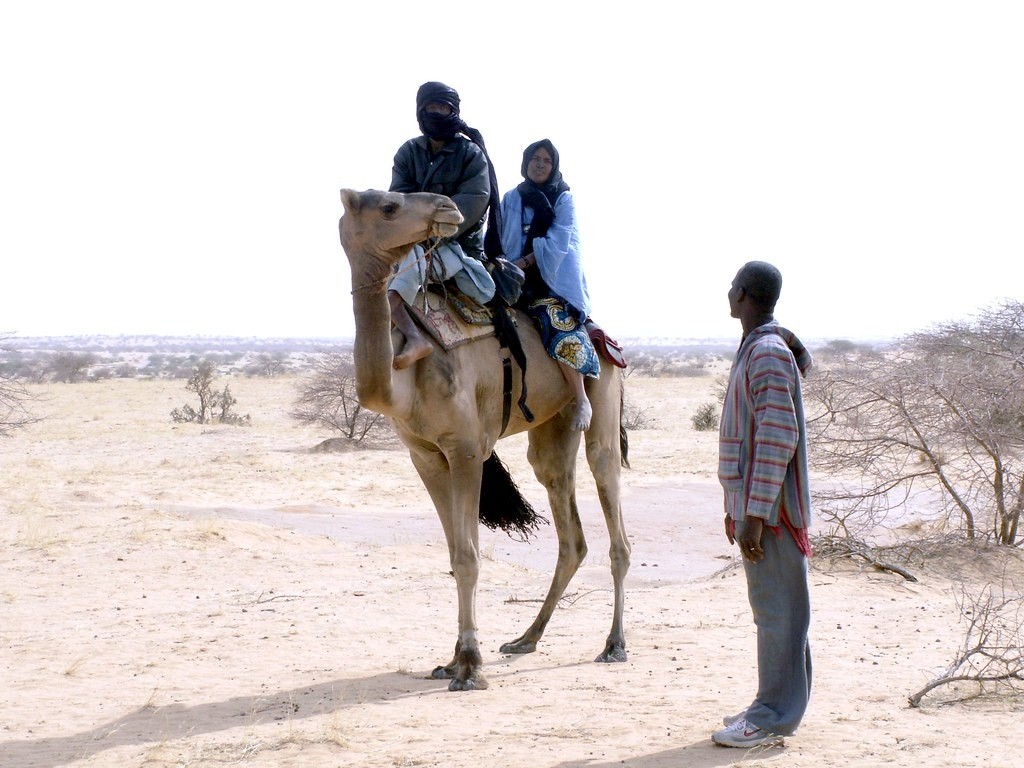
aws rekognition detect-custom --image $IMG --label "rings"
[750,548,754,550]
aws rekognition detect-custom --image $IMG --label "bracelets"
[521,256,531,266]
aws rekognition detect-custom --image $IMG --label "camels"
[339,185,632,692]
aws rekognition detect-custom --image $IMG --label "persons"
[386,82,491,370]
[710,261,813,746]
[481,138,601,432]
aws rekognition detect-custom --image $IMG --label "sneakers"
[711,716,777,748]
[721,706,797,737]
